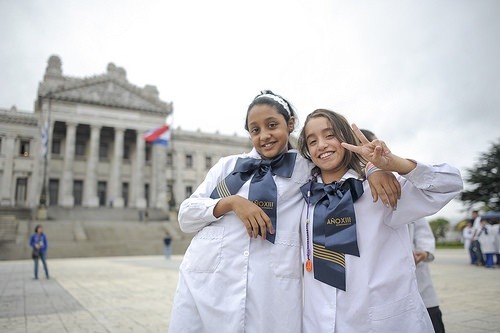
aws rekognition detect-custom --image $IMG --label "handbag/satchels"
[32,249,40,259]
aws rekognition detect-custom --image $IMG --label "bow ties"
[299,176,365,292]
[208,152,297,243]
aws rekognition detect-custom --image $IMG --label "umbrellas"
[479,211,500,221]
[455,217,474,230]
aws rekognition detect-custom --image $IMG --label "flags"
[145,125,171,146]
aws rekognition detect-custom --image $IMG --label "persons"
[29,224,49,280]
[164,89,500,333]
